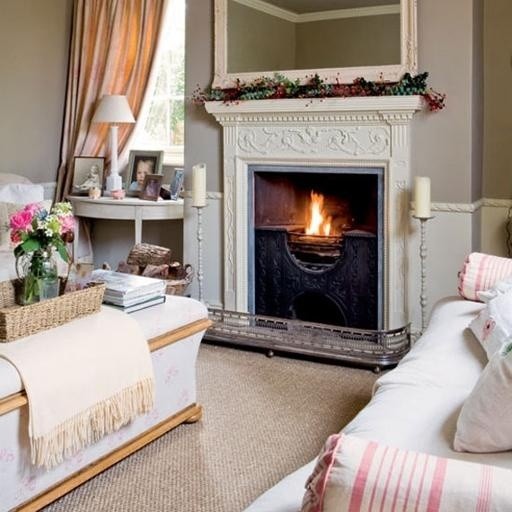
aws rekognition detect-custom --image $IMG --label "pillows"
[449,247,512,301]
[460,283,512,363]
[295,427,512,512]
[448,331,512,455]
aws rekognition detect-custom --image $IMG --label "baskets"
[103,262,194,296]
[0,276,106,343]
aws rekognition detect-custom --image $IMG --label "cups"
[36,276,60,303]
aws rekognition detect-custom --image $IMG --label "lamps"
[90,92,139,198]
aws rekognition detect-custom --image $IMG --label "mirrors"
[210,0,420,91]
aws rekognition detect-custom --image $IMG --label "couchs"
[226,243,512,512]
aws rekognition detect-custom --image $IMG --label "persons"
[129,156,156,193]
[143,178,157,197]
[76,163,100,194]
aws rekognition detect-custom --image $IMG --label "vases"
[23,246,63,306]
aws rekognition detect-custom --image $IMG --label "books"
[85,268,167,313]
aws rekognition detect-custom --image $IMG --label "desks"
[60,191,186,248]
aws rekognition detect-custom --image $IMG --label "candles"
[192,161,206,207]
[413,176,431,217]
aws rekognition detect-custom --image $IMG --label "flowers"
[6,195,78,302]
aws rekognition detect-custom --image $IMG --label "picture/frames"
[125,150,164,199]
[70,156,107,196]
[166,168,186,200]
[138,175,165,203]
